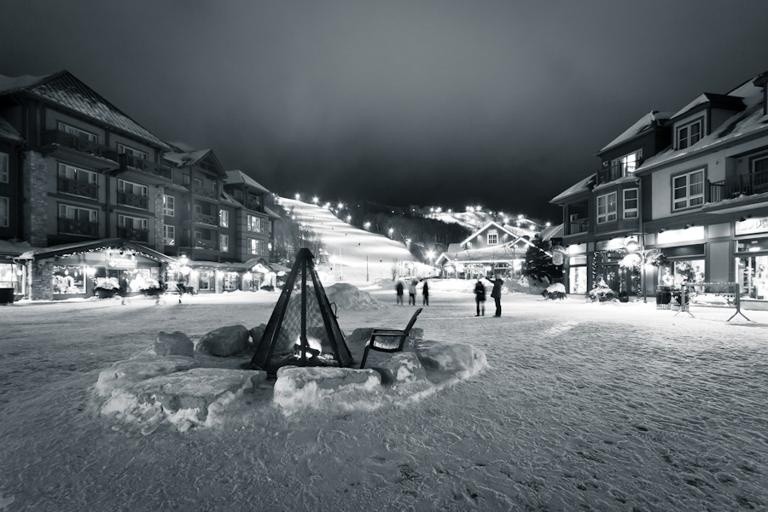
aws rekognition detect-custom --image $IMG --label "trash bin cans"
[656,286,671,309]
[182,286,193,294]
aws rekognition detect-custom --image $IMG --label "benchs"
[360,308,422,370]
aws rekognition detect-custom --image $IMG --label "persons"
[422,282,429,305]
[486,276,504,317]
[395,280,405,304]
[473,280,487,316]
[409,280,417,305]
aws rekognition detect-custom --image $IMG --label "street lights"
[620,231,661,304]
